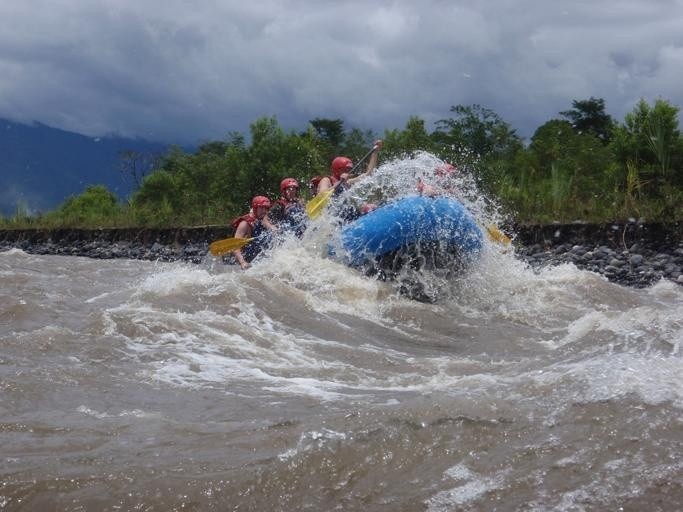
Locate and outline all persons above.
[229,139,461,272]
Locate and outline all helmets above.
[252,196,271,207]
[311,176,322,185]
[435,164,454,176]
[332,156,353,172]
[280,178,299,191]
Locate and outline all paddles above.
[456,193,512,247]
[211,221,305,256]
[306,145,378,221]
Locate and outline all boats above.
[241,194,484,304]
